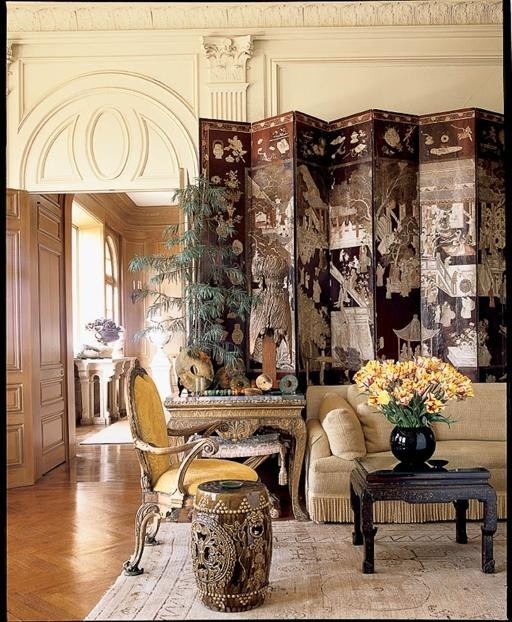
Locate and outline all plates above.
[427,460,449,470]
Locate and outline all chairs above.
[122,364,261,579]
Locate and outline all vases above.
[389,425,437,469]
[93,331,120,358]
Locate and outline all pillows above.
[318,389,391,461]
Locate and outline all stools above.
[192,433,286,518]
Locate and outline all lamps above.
[131,280,143,304]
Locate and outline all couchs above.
[307,385,507,524]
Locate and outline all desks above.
[349,456,495,575]
[76,359,136,424]
[165,389,307,525]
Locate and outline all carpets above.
[86,523,508,621]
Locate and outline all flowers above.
[88,317,121,343]
[352,358,475,426]
